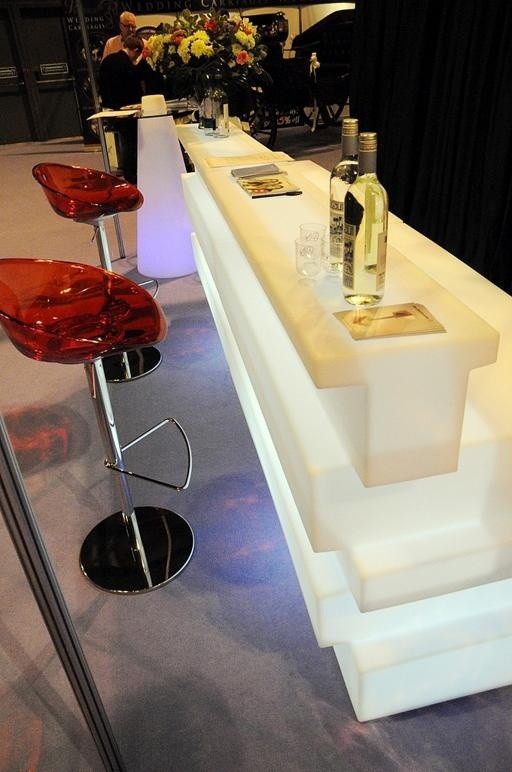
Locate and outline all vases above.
[193,89,215,134]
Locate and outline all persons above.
[98,36,151,184]
[103,12,150,179]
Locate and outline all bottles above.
[213,67,229,138]
[198,96,204,129]
[329,118,359,269]
[343,131,389,309]
[205,78,217,136]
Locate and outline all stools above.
[0,256,196,596]
[32,159,165,384]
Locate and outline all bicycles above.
[237,41,348,150]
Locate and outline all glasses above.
[120,22,136,28]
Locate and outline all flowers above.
[140,10,262,89]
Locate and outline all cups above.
[294,239,322,278]
[320,231,335,273]
[299,223,326,258]
